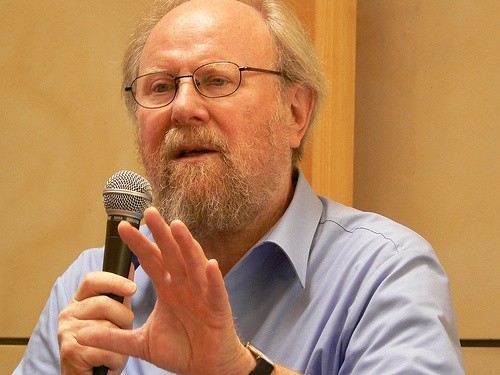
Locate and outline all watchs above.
[244,341,275,375]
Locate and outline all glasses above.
[125,61,282,109]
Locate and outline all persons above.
[10,1,467,375]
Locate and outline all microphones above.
[93,170,154,375]
